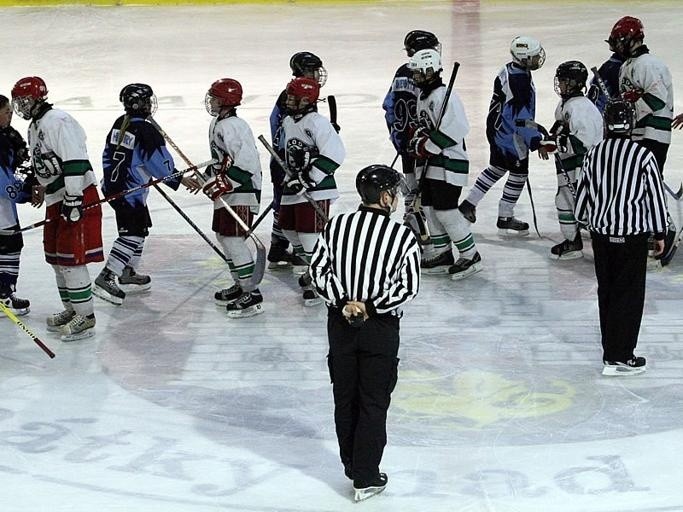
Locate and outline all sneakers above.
[458,198,477,224]
[448,249,482,275]
[297,270,321,304]
[60,311,96,336]
[0,293,30,311]
[214,283,245,302]
[421,248,455,268]
[226,286,264,311]
[602,355,647,368]
[352,471,389,490]
[94,265,127,300]
[266,240,306,266]
[118,265,151,286]
[551,230,584,255]
[496,216,530,231]
[46,308,77,327]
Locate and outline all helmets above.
[204,78,243,118]
[284,77,321,116]
[355,163,413,204]
[402,28,442,59]
[290,51,328,89]
[603,98,633,139]
[405,47,444,76]
[508,35,548,71]
[9,76,49,121]
[119,81,158,120]
[553,59,589,98]
[604,15,645,58]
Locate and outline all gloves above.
[201,171,235,203]
[60,191,83,225]
[539,135,560,155]
[281,169,318,198]
[401,121,439,160]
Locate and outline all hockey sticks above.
[0,158,219,236]
[408,62,460,242]
[526,178,541,238]
[147,115,266,285]
[662,181,682,200]
[514,118,576,203]
[242,95,340,241]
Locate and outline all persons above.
[573,99,669,368]
[402,46,481,275]
[10,76,104,336]
[308,164,421,489]
[382,30,443,246]
[539,16,683,267]
[0,94,41,311]
[277,79,346,300]
[93,83,201,299]
[184,78,264,312]
[266,52,341,267]
[458,35,546,231]
